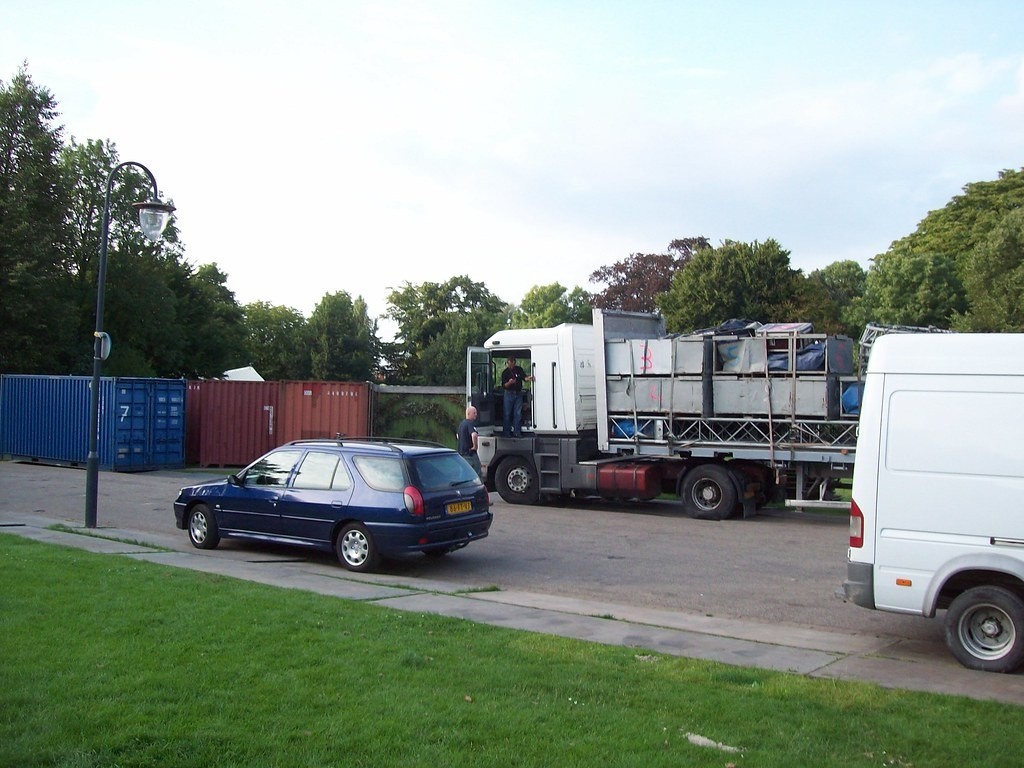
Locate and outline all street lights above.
[86,162,176,528]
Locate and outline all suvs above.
[173,434,490,571]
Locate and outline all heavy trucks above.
[464,306,867,519]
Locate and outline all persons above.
[456,406,493,506]
[501,357,535,438]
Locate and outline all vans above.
[834,332,1024,670]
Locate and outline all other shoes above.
[505,434,511,438]
[514,434,524,438]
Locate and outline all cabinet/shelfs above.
[606,322,866,418]
